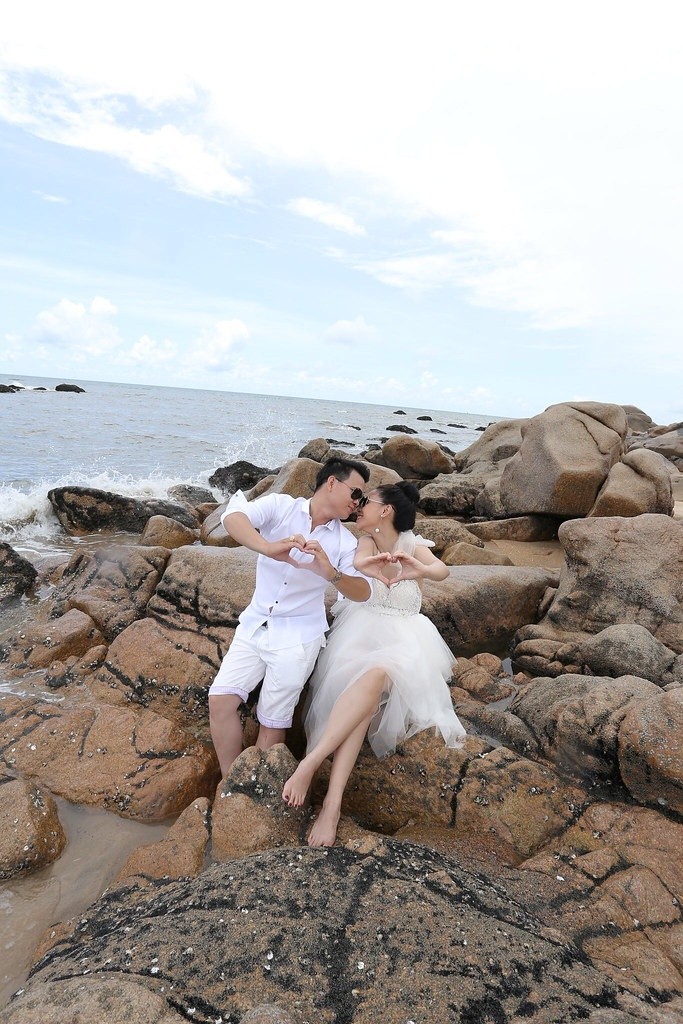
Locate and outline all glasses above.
[335,478,370,500]
[358,495,397,513]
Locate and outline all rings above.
[320,548,323,553]
[290,535,295,542]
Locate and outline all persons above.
[282,479,466,848]
[208,459,374,777]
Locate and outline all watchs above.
[328,568,341,583]
